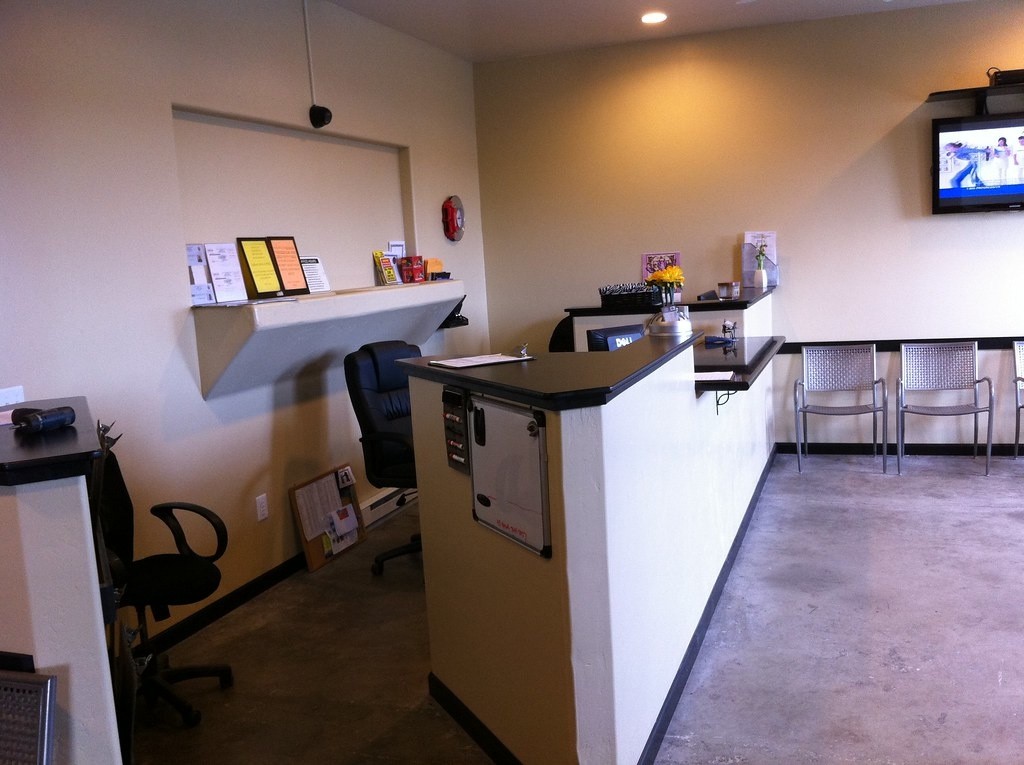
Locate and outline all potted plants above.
[659,308,679,320]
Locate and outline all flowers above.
[645,265,684,306]
[755,234,768,271]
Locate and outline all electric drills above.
[7,406,76,435]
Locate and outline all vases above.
[754,270,767,289]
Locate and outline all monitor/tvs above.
[932,111,1024,215]
[697,289,719,300]
[587,323,645,352]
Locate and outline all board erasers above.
[473,406,486,446]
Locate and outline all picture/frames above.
[642,250,679,284]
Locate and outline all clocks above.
[442,195,466,240]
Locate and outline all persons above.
[1013,136,1024,178]
[945,142,991,188]
[993,137,1011,185]
[983,146,990,161]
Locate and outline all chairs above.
[897,342,995,477]
[344,340,421,574]
[1013,341,1024,458]
[88,450,236,728]
[794,344,889,474]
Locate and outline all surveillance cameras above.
[309,104,332,128]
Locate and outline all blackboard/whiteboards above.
[463,391,555,559]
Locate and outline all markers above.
[447,438,465,451]
[447,452,465,463]
[442,412,463,423]
